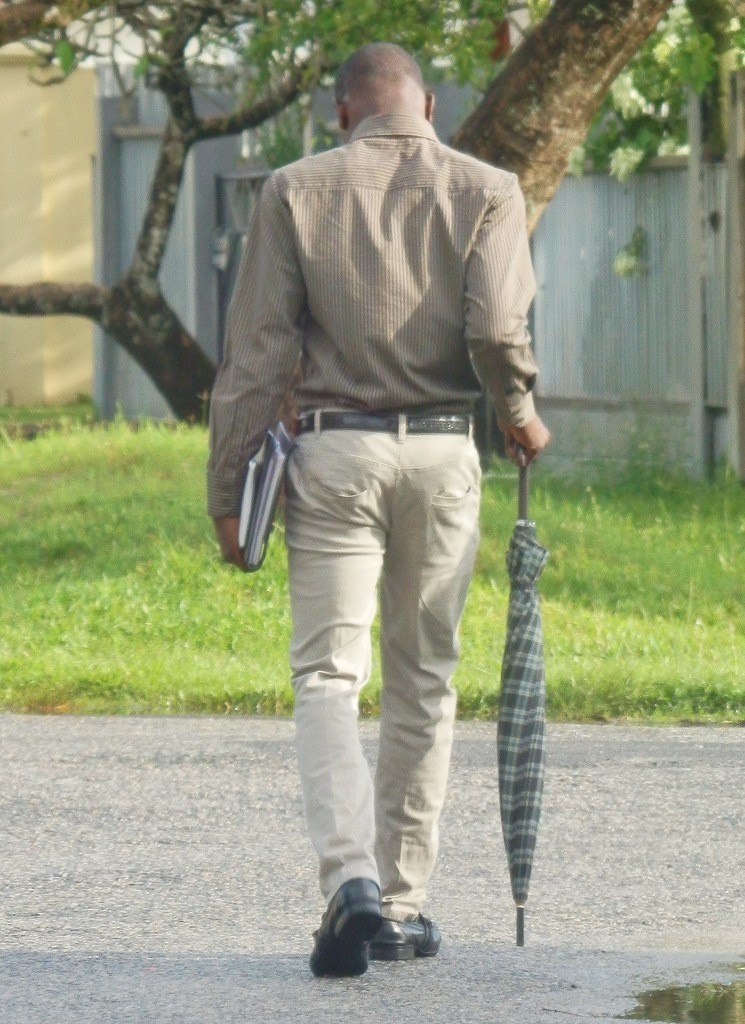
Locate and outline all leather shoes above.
[309,879,382,978]
[369,912,441,961]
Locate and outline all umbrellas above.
[496,436,546,946]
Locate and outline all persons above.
[205,43,552,978]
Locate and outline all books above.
[234,419,295,575]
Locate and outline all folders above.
[245,421,293,573]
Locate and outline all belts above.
[296,412,470,434]
